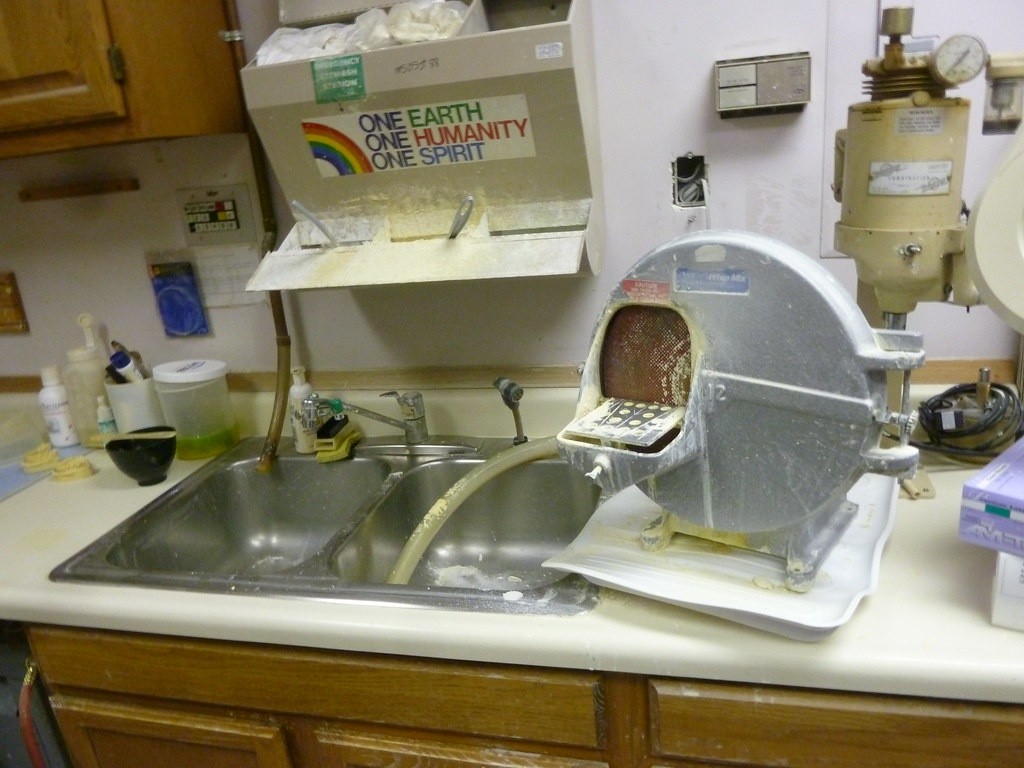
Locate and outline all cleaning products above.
[290,366,324,454]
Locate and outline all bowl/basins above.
[106,426,176,486]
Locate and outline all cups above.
[103,377,165,434]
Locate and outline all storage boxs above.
[991,551,1024,631]
[959,435,1024,556]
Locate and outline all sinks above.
[109,460,388,580]
[302,390,430,445]
[334,461,611,616]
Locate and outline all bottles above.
[152,358,238,461]
[96,396,117,434]
[38,367,78,447]
[62,312,106,447]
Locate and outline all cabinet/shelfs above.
[0,0,247,159]
[17,626,1024,768]
